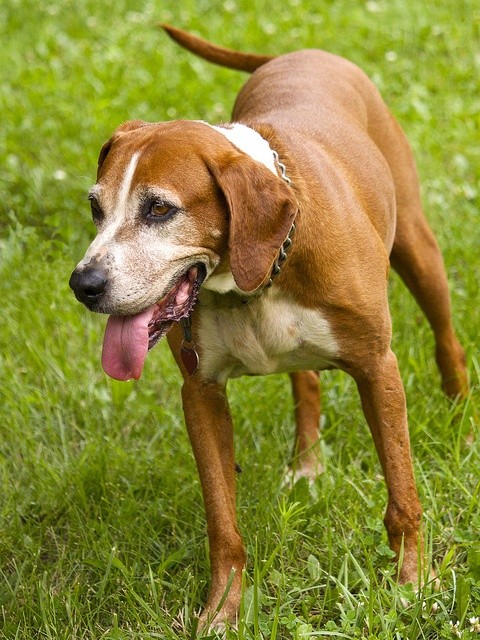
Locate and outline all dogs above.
[70,24,480,640]
